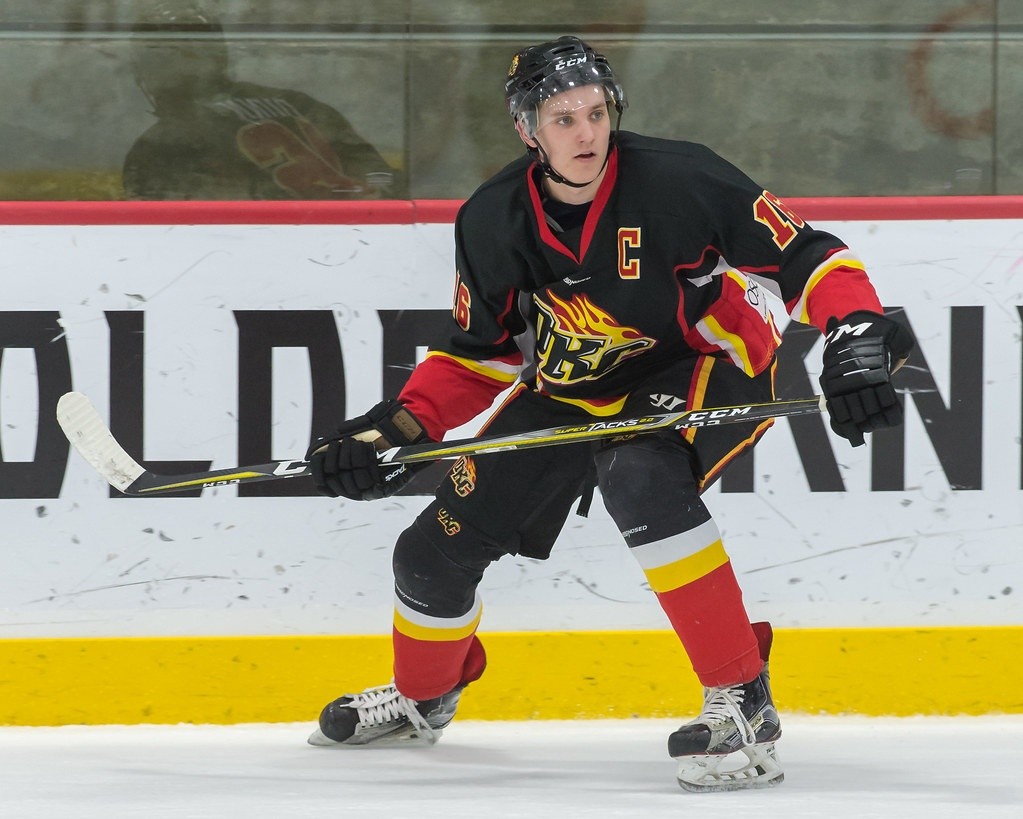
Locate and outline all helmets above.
[132,0,228,111]
[504,35,624,157]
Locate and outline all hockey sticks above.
[53,385,825,501]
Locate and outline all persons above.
[120,0,408,198]
[320,36,900,758]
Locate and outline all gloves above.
[818,309,914,447]
[305,396,440,501]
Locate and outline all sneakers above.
[306,634,486,748]
[667,621,784,793]
